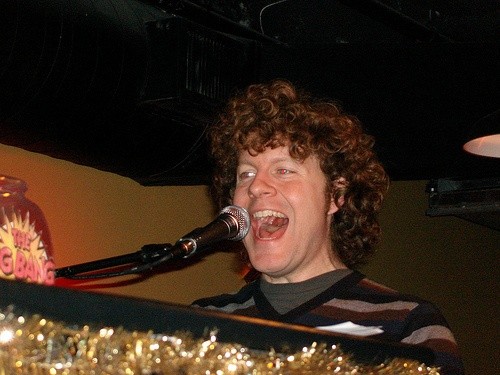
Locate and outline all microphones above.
[170,205,251,258]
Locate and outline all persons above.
[181,75,467,375]
